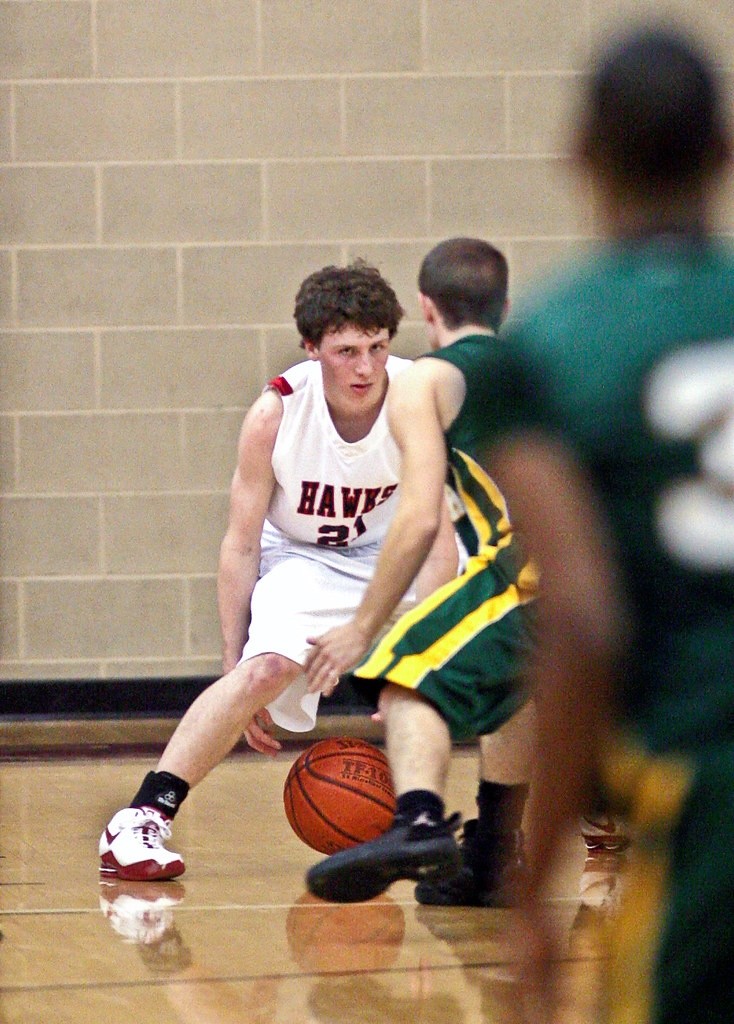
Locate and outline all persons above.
[90,877,625,1023]
[300,238,547,907]
[482,25,733,1024]
[95,258,633,880]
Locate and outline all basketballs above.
[279,737,400,855]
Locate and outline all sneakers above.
[98,806,185,882]
[99,881,186,947]
[305,811,463,903]
[415,820,529,909]
[570,816,638,946]
